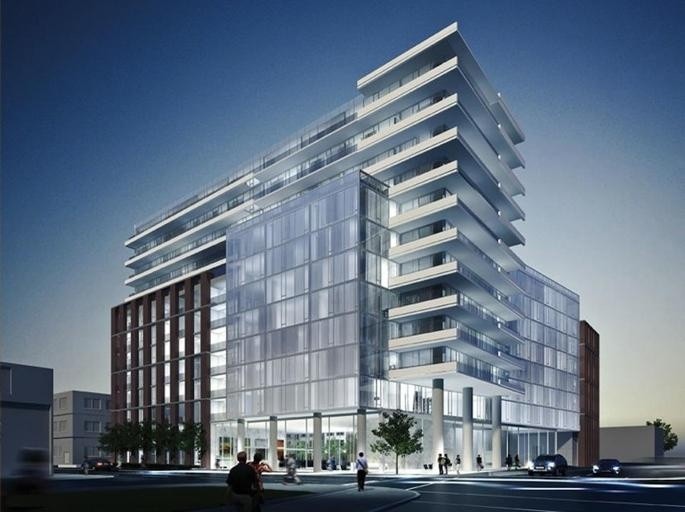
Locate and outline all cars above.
[590,458,624,476]
[78,457,117,475]
[524,454,571,476]
